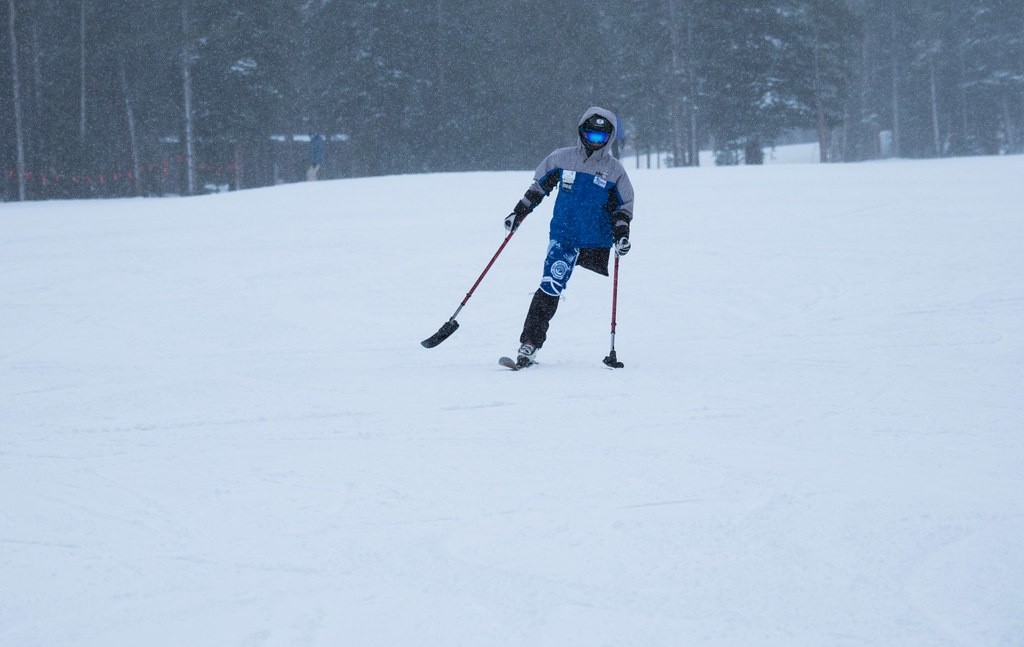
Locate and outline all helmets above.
[580,115,613,150]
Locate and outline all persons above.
[502,106,635,365]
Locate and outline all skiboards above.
[498,355,538,371]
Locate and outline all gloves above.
[503,210,525,233]
[614,237,631,257]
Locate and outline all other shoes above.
[517,341,539,363]
